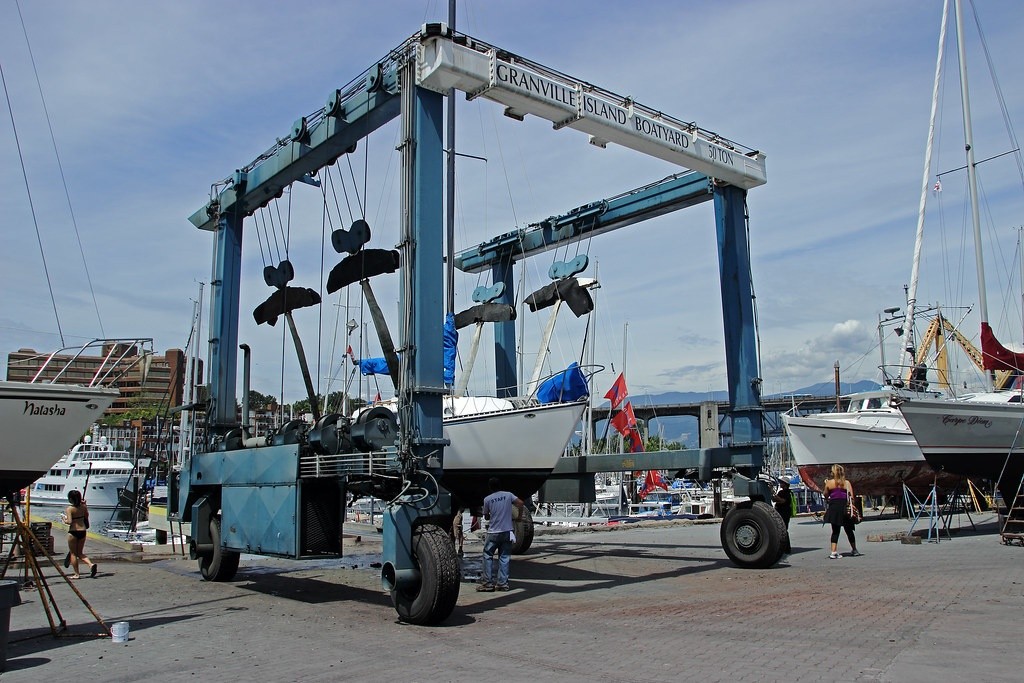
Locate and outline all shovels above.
[470,507,543,525]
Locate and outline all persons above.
[62,490,97,578]
[772,482,794,530]
[475,477,523,592]
[453,510,464,557]
[9,501,26,523]
[821,464,860,558]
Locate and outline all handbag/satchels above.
[846,504,862,524]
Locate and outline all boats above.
[775,304,980,503]
[20,431,146,509]
[1,332,158,507]
[537,481,714,520]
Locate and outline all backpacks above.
[788,490,796,516]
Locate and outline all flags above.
[603,371,628,409]
[639,470,668,498]
[610,400,637,438]
[629,431,648,477]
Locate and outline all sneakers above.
[851,549,859,555]
[829,552,842,559]
[476,583,495,592]
[494,584,509,591]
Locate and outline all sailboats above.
[883,0,1024,503]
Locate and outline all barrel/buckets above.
[111,622,129,643]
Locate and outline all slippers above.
[90,564,97,577]
[69,575,80,579]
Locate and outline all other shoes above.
[458,549,463,556]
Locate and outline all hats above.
[777,477,790,487]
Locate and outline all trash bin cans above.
[851,497,863,517]
[0,580,18,672]
[156,529,167,544]
